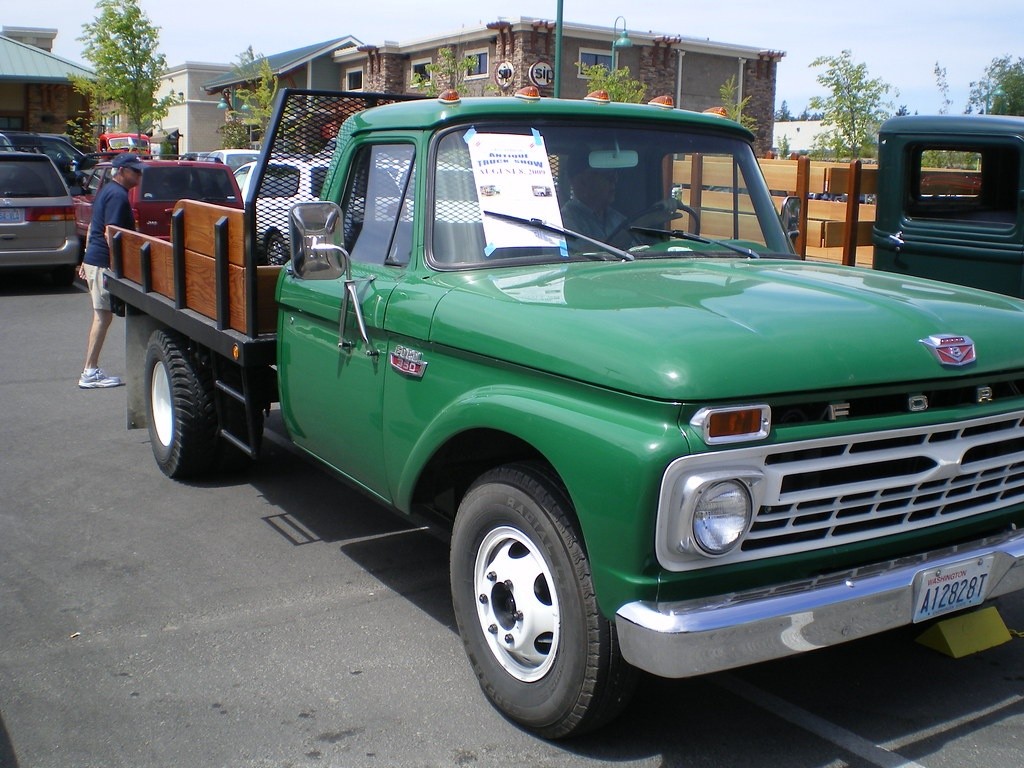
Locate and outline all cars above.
[378,161,482,224]
[0,150,81,286]
[187,149,261,191]
[232,158,407,266]
[99,133,151,161]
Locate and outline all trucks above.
[102,86,1024,743]
[671,115,1024,302]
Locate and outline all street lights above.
[612,16,632,70]
[217,89,250,120]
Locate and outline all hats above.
[112,153,150,170]
[567,134,622,178]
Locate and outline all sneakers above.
[78,368,121,388]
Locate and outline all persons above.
[79,153,152,388]
[542,137,684,255]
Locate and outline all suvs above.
[1,130,93,195]
[70,154,244,241]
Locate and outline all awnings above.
[150,128,178,143]
